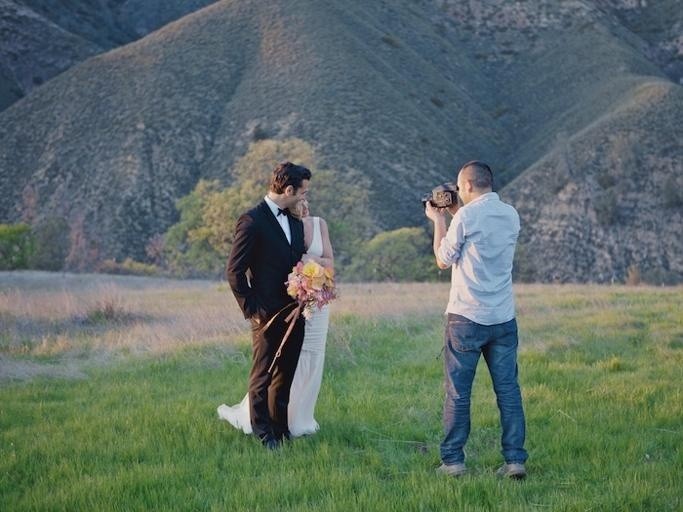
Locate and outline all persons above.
[217,198,334,439]
[226,160,312,453]
[425,160,529,477]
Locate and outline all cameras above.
[422,184,458,209]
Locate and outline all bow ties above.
[276,207,290,217]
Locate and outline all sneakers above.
[496,462,528,479]
[437,462,469,476]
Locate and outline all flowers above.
[284,257,339,322]
